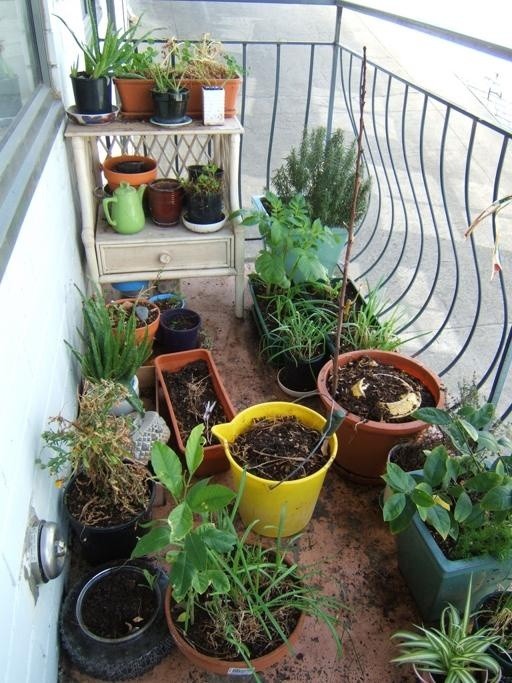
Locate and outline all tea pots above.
[102,180,149,234]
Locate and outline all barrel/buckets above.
[211,393,338,539]
